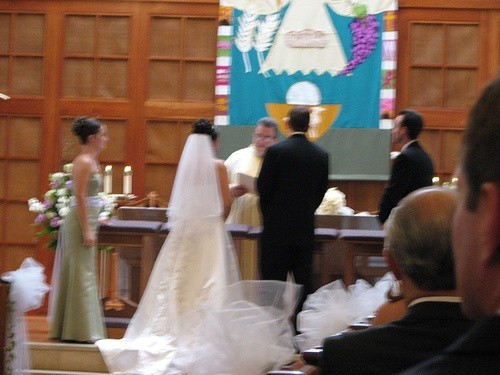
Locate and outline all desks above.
[99,219,384,307]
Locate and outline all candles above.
[124,166,132,195]
[103,164,113,195]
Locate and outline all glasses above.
[252,133,277,142]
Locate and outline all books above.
[237,157,263,196]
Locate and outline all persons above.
[48,119,107,342]
[223,118,279,305]
[300,76,500,375]
[254,106,330,354]
[161,120,234,342]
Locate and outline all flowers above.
[28,163,119,253]
